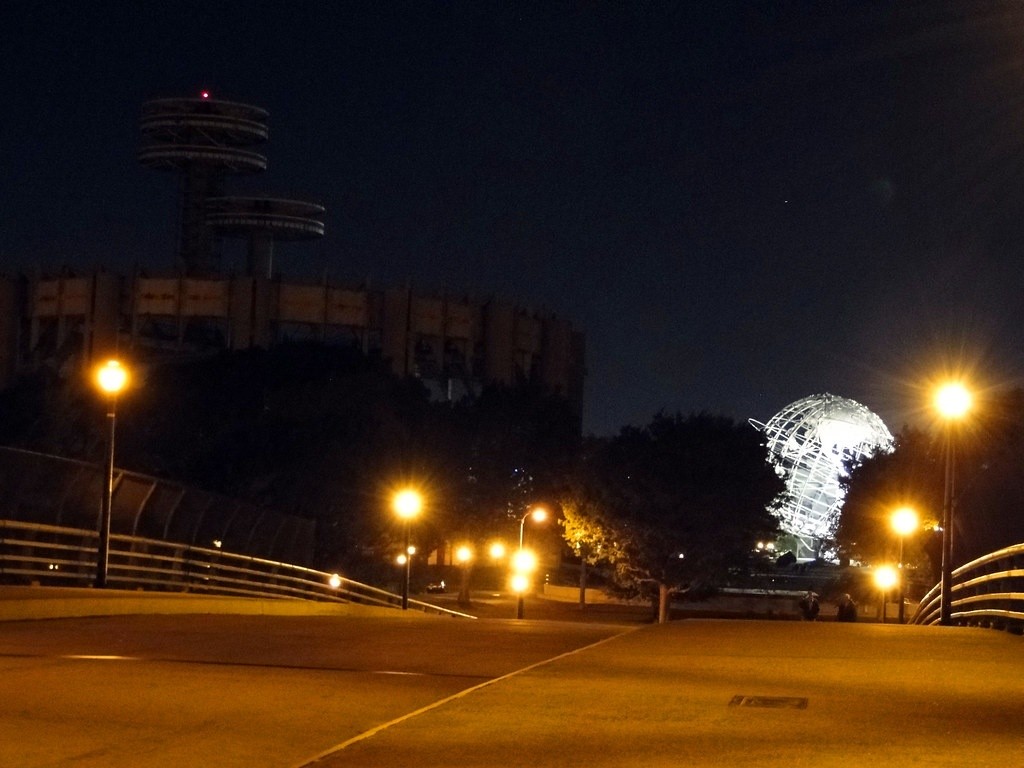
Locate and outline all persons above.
[834,593,857,623]
[799,590,820,621]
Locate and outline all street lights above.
[877,566,897,623]
[934,381,971,629]
[93,359,129,588]
[891,507,918,625]
[394,488,420,610]
[516,506,547,621]
